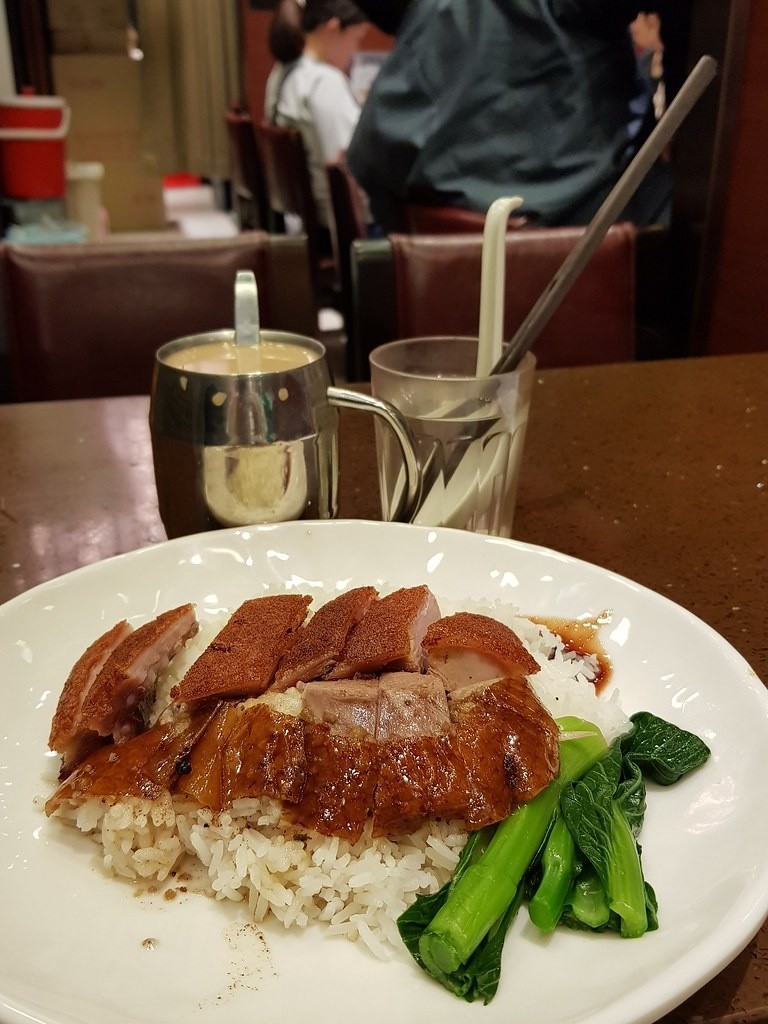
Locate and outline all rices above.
[45,581,638,957]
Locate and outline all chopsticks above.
[393,56,717,522]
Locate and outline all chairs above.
[224,109,363,232]
[0,233,281,403]
[389,218,641,374]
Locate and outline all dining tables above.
[0,350,768,1024]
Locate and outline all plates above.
[0,520,768,1024]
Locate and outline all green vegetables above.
[395,706,707,1007]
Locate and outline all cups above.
[363,333,537,543]
[66,158,103,248]
[151,324,423,546]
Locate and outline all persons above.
[258,0,703,384]
